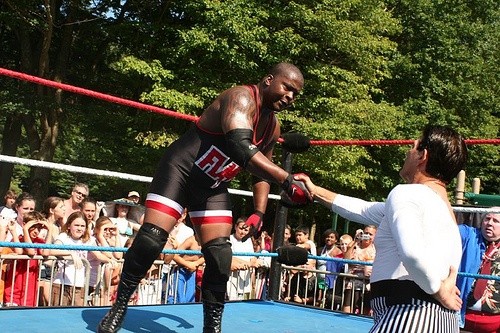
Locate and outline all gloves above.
[281,173,314,205]
[241,211,265,242]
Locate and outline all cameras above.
[357,233,370,241]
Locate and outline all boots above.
[98,279,137,332]
[202,301,224,333]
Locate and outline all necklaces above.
[427,181,446,188]
[482,250,499,261]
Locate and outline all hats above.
[112,198,140,208]
[128,191,140,197]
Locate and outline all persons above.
[98,63,314,333]
[0,183,500,333]
[288,126,466,333]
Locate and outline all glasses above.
[75,191,86,198]
